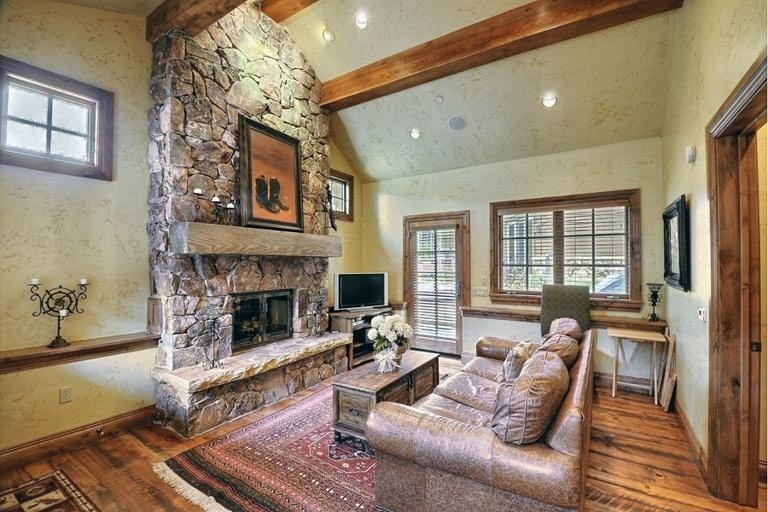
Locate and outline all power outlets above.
[58,385,73,407]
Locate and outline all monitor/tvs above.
[333,271,389,312]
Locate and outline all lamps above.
[645,282,665,322]
[538,87,557,112]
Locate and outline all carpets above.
[152,346,468,512]
[0,468,104,511]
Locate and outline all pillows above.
[490,348,570,447]
[364,326,596,511]
[546,316,583,341]
[495,337,530,385]
[533,333,579,370]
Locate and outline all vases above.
[387,340,412,372]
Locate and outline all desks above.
[605,327,666,406]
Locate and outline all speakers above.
[353,329,372,343]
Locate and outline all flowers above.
[366,316,412,373]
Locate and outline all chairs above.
[539,283,591,339]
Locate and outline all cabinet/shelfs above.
[231,289,295,354]
[327,306,408,370]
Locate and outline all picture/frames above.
[659,194,690,293]
[236,113,304,233]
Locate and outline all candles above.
[59,309,69,321]
[78,278,89,289]
[30,275,41,286]
